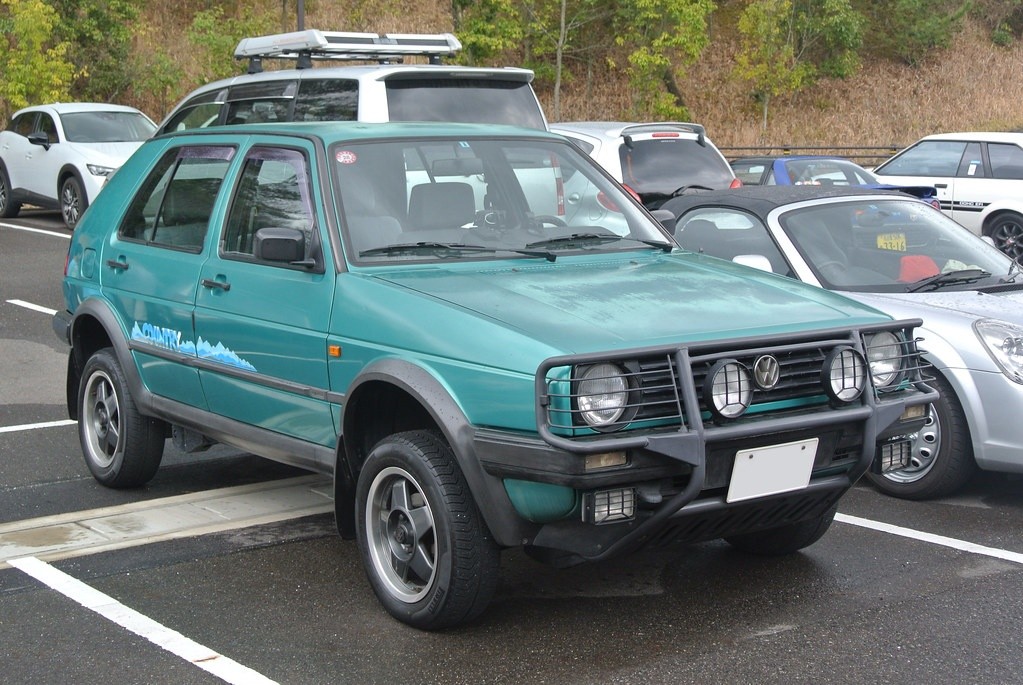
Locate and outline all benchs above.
[685,220,790,272]
[163,179,304,257]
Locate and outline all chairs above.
[407,182,477,244]
[344,170,403,254]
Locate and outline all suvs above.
[794,131,1023,265]
[50,119,941,631]
[101,28,565,225]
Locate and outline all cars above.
[0,101,160,230]
[728,154,940,251]
[546,121,752,236]
[628,185,1023,500]
